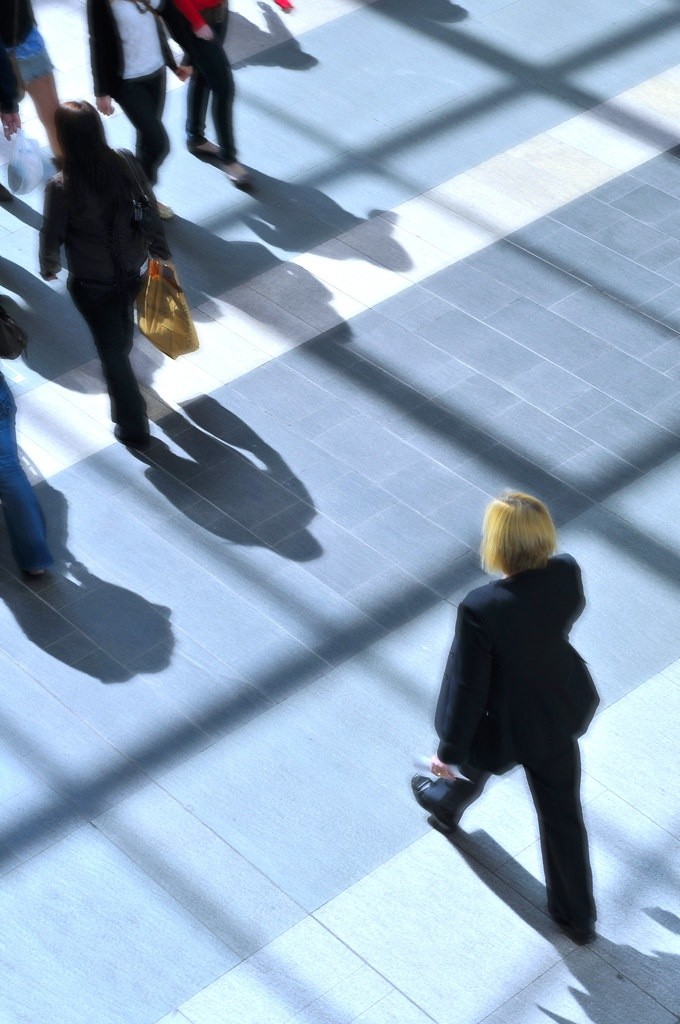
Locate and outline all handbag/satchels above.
[3,121,43,197]
[0,307,28,361]
[134,259,199,359]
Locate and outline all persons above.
[0,0,260,579]
[411,490,600,945]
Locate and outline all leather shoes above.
[548,901,596,946]
[412,774,457,832]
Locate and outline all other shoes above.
[113,425,150,451]
[187,140,223,155]
[157,204,173,221]
[224,163,249,187]
[26,568,46,577]
